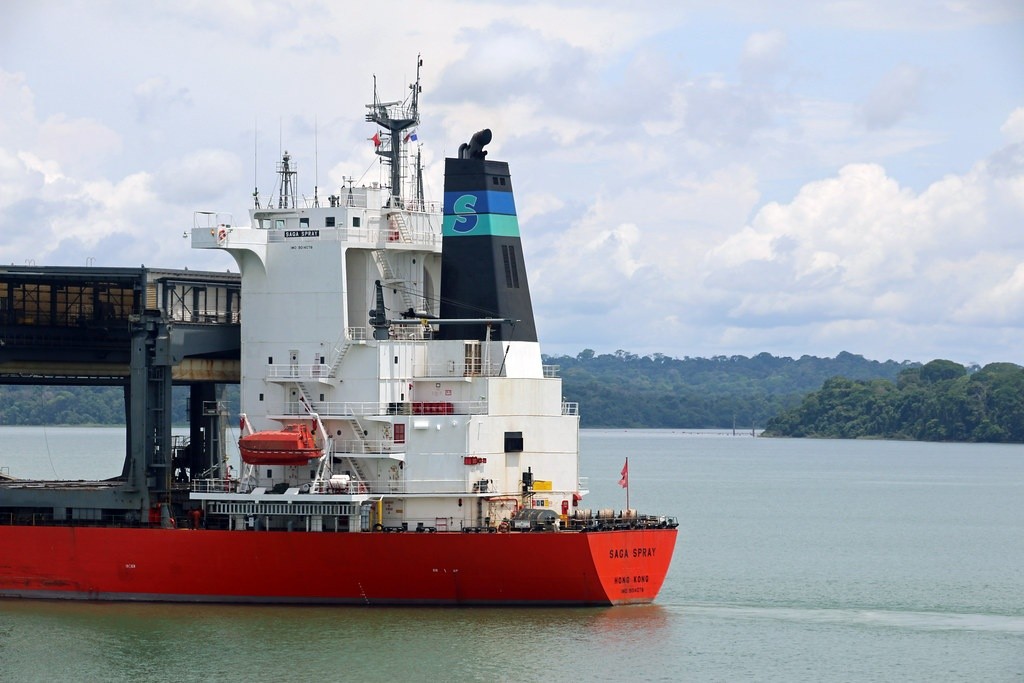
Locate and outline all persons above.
[188,507,201,530]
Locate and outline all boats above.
[0,54,679,606]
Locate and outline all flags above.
[371,133,379,147]
[618,461,628,489]
[403,129,417,144]
